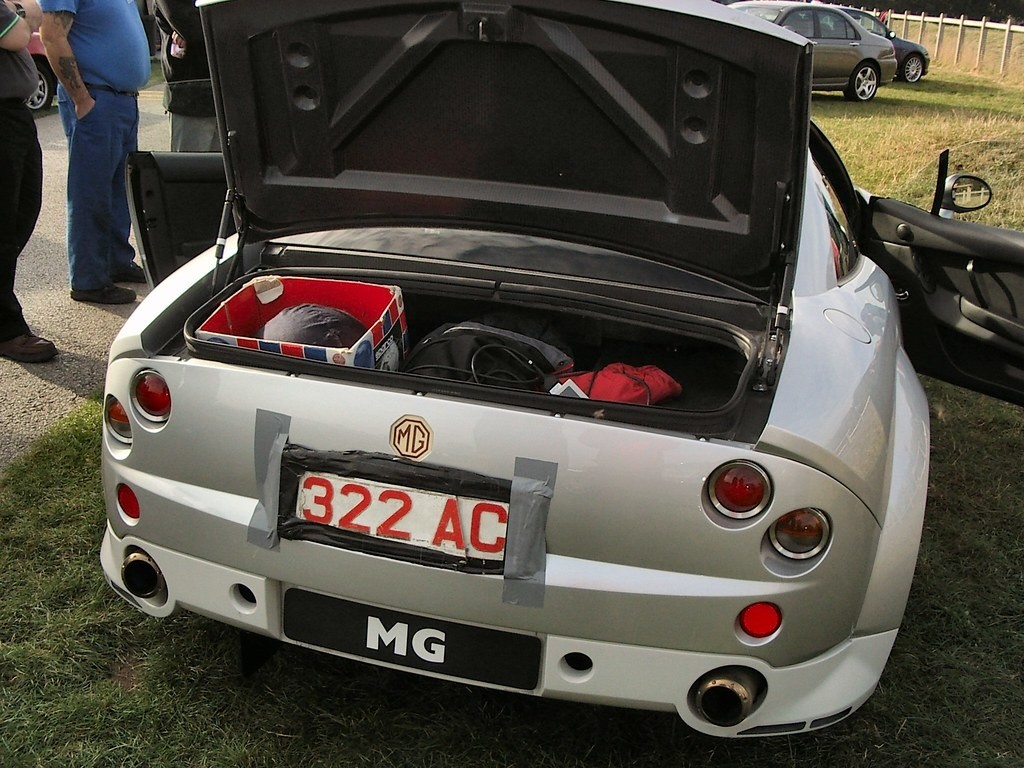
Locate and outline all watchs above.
[14,2,25,18]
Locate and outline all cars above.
[804,5,931,83]
[26,30,59,111]
[725,1,898,101]
[98,0,1024,740]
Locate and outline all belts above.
[0,97,31,105]
[85,83,139,97]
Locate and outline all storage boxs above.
[195,275,409,373]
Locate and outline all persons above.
[0,0,56,364]
[152,0,222,150]
[38,0,150,304]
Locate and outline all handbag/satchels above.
[401,321,573,395]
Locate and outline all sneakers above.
[71,283,136,303]
[0,331,59,363]
[109,262,146,283]
